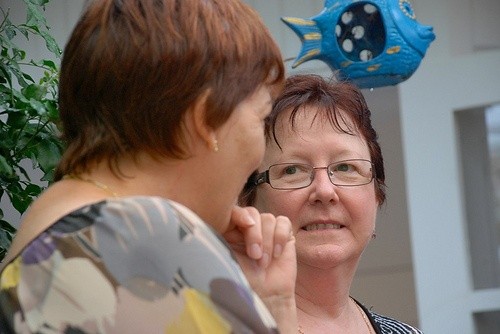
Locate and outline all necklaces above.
[61,174,124,199]
[297,297,372,333]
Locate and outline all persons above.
[240,69,423,334]
[0,0,298,333]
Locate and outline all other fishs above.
[280,0,435,89]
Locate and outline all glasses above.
[255,159,377,190]
